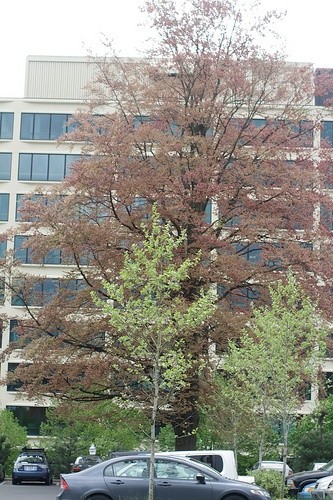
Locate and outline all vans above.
[117,450,238,483]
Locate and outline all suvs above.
[11,447,54,485]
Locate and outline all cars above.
[284,458,333,498]
[70,455,103,473]
[56,454,270,500]
[251,461,294,477]
[298,475,333,500]
